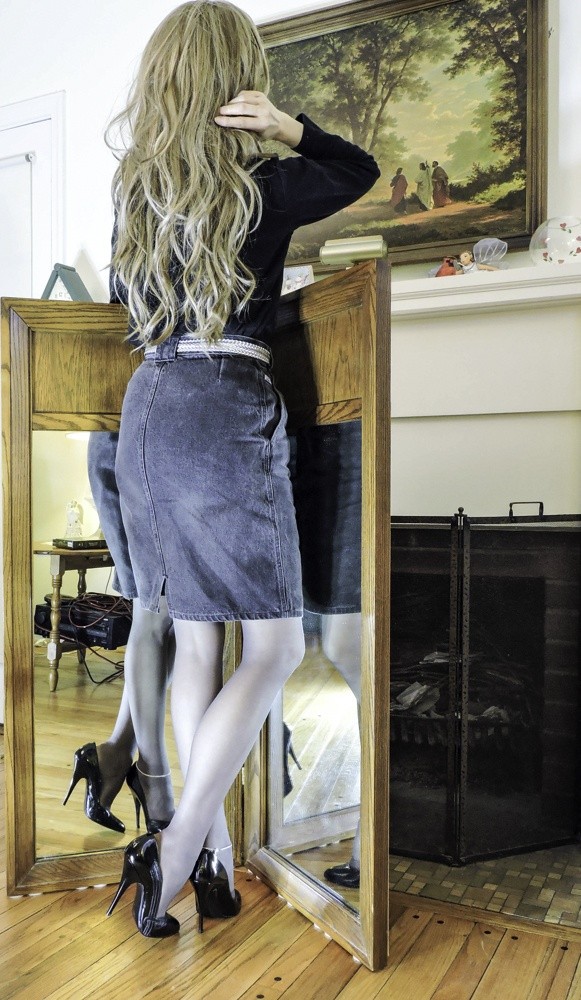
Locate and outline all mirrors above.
[29,414,233,864]
[266,619,361,855]
[251,413,366,915]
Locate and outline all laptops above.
[53,537,110,549]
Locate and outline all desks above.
[33,540,111,692]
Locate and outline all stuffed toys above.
[455,249,500,276]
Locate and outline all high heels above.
[126,760,170,834]
[104,834,180,936]
[323,867,360,886]
[63,742,126,833]
[193,848,242,934]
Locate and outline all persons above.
[62,430,176,834]
[106,0,380,938]
[283,423,363,887]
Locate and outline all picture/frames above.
[157,1,550,273]
